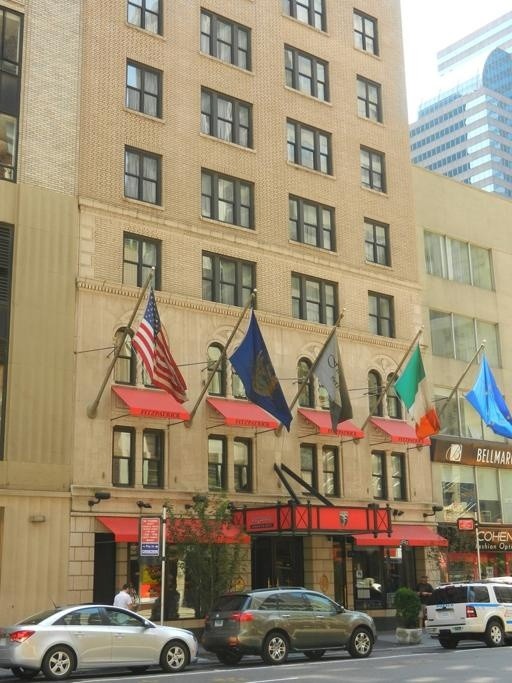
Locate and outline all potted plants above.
[394,587,422,646]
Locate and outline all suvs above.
[423,576,512,648]
[202,586,377,667]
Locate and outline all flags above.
[226,309,295,433]
[313,323,354,433]
[395,343,441,441]
[462,356,511,439]
[133,292,188,402]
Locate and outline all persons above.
[112,583,135,612]
[148,598,168,620]
[481,571,486,578]
[163,583,180,618]
[415,575,434,629]
[127,582,140,611]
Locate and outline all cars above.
[0,598,201,680]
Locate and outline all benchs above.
[57,612,111,625]
